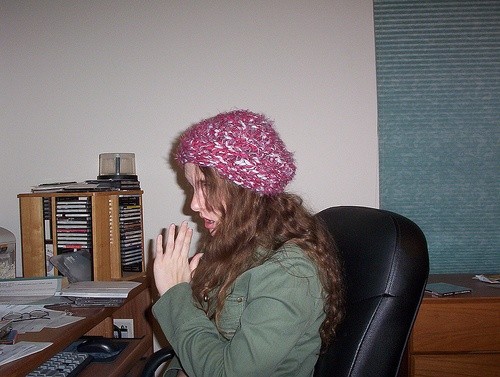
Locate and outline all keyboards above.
[25,352,92,377]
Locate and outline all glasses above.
[1,310,51,322]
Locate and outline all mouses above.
[76,338,117,354]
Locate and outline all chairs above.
[142,206,429,377]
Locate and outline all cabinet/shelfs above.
[0,276,154,377]
[406,273,500,377]
[17,190,145,282]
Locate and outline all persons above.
[153,110,346,377]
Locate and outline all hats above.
[171,110,296,197]
